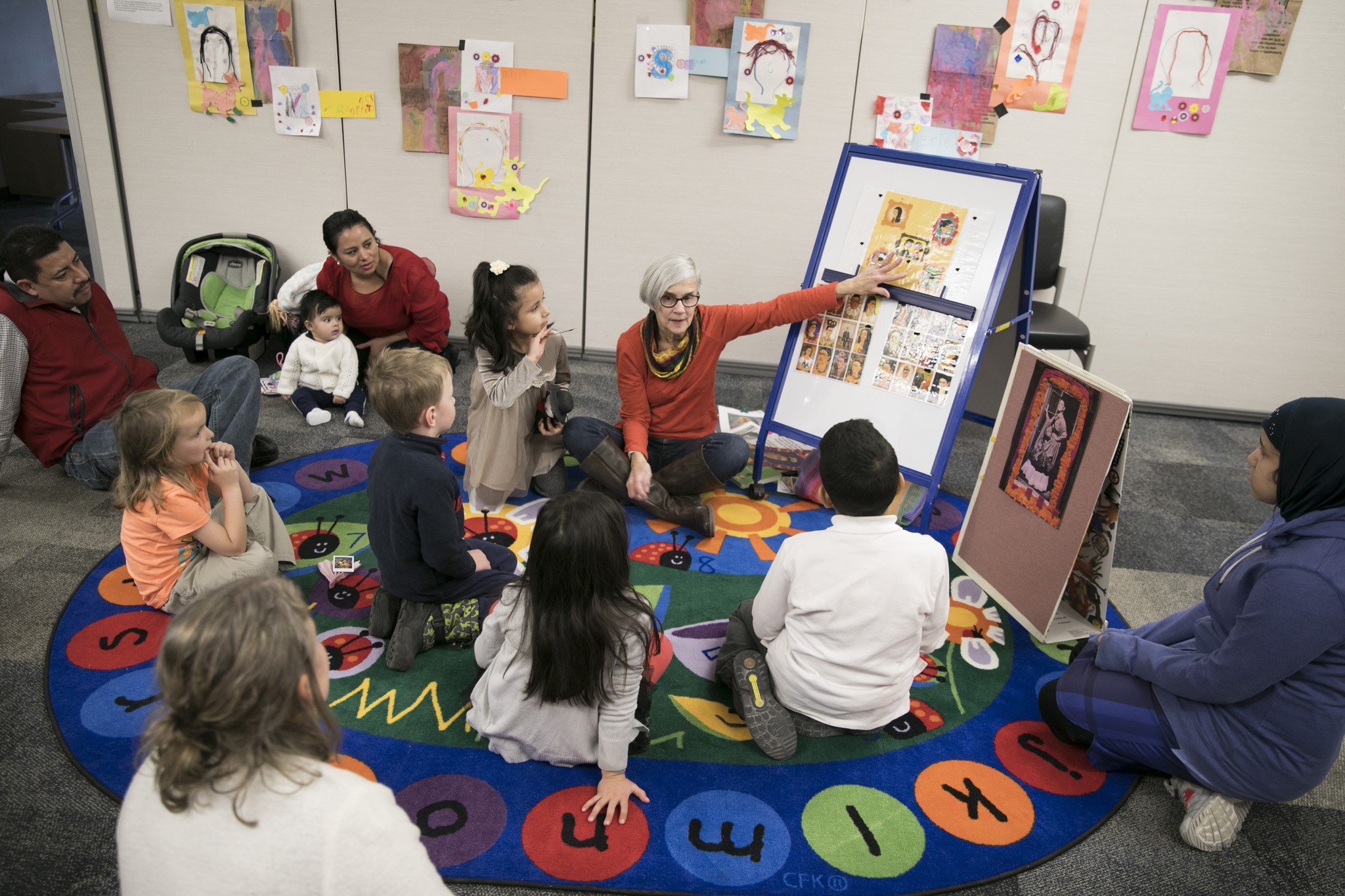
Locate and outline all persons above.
[466,490,662,825]
[714,419,950,759]
[116,578,453,896]
[278,289,366,427]
[367,346,519,670]
[563,249,907,538]
[316,208,451,390]
[0,225,279,491]
[1040,397,1345,852]
[465,261,571,506]
[1021,399,1067,492]
[114,388,295,613]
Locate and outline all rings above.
[878,263,882,268]
[871,262,875,265]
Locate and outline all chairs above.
[1028,194,1096,373]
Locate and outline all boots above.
[385,596,488,671]
[653,445,726,496]
[579,435,715,538]
[368,582,403,639]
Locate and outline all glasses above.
[659,292,700,307]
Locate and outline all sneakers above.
[251,435,278,467]
[734,650,797,760]
[1163,777,1254,853]
[789,708,883,738]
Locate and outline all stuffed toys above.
[535,385,574,435]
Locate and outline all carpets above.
[48,432,1142,896]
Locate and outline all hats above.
[1261,398,1298,451]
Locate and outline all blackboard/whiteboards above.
[770,142,1044,486]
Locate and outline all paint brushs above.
[544,328,576,337]
